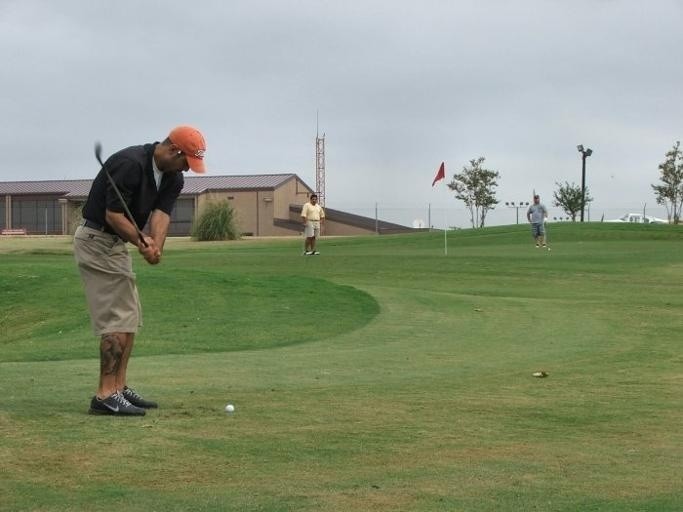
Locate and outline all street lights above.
[578,145,593,222]
[504,201,529,223]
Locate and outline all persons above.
[299,194,326,255]
[70,124,208,418]
[526,194,549,249]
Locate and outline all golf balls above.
[548,247,552,250]
[226,403,234,412]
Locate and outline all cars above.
[605,214,667,224]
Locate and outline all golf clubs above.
[95,143,149,248]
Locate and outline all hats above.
[168,125,206,174]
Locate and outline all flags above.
[431,162,446,187]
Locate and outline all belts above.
[79,218,115,234]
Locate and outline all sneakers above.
[123,386,158,408]
[305,250,320,255]
[536,243,546,248]
[88,392,145,416]
